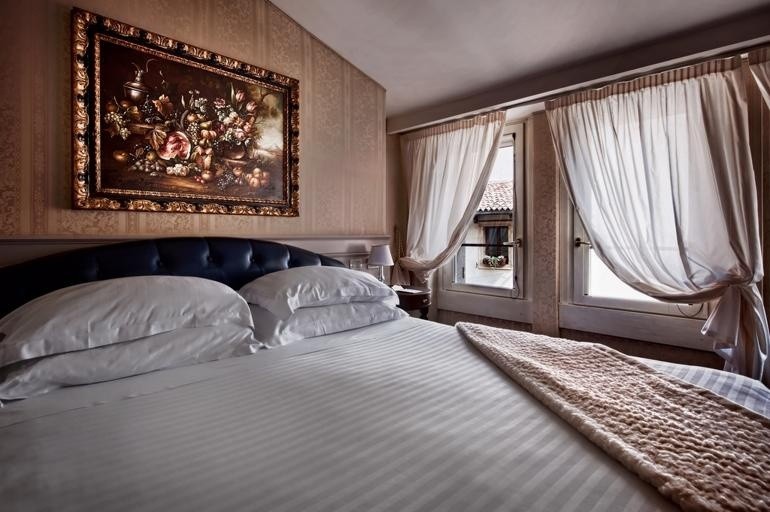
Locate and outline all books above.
[397,287,424,294]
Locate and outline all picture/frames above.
[69,2,302,219]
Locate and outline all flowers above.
[139,82,259,177]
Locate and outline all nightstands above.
[388,284,432,320]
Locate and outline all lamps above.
[365,243,398,286]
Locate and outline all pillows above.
[249,299,410,351]
[0,323,264,409]
[0,274,257,368]
[237,266,397,323]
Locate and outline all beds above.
[0,233,770,512]
